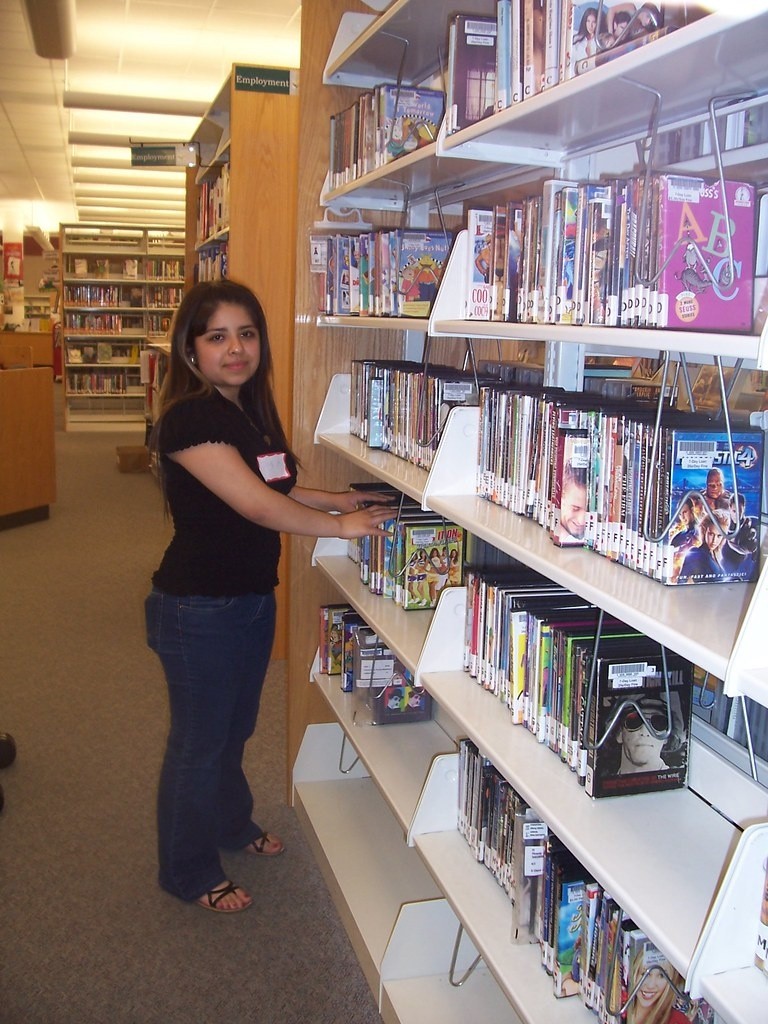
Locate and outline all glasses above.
[623,709,668,732]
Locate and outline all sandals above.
[248,831,285,856]
[196,879,254,911]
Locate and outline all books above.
[305,2,768,1024]
[0,152,236,433]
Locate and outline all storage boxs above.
[116,446,147,475]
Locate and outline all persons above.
[668,491,703,552]
[676,508,730,584]
[408,550,418,605]
[415,548,429,607]
[447,537,460,586]
[142,279,401,912]
[604,689,685,773]
[434,546,450,602]
[571,7,606,60]
[558,459,587,542]
[422,547,446,607]
[573,1,659,40]
[719,492,758,578]
[631,943,692,1024]
[596,11,632,52]
[698,466,735,520]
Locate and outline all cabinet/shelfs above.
[59,222,186,434]
[0,293,55,532]
[284,1,768,1024]
[184,63,300,663]
[144,336,172,429]
